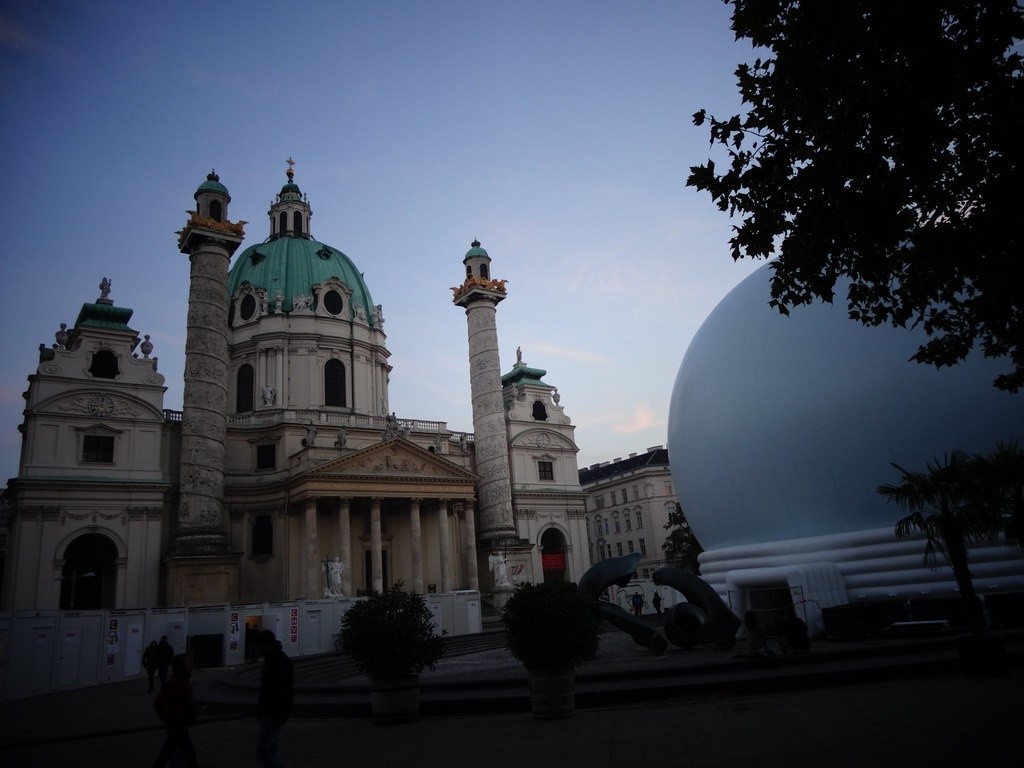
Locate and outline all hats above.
[256,630,276,648]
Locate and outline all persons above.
[385,412,398,436]
[516,346,522,362]
[653,591,663,617]
[55,323,69,345]
[99,277,113,298]
[142,635,174,694]
[632,592,644,616]
[338,425,349,448]
[497,551,510,585]
[141,334,153,355]
[263,384,276,404]
[154,652,201,768]
[332,557,341,596]
[304,420,318,446]
[254,629,296,764]
[433,436,442,449]
